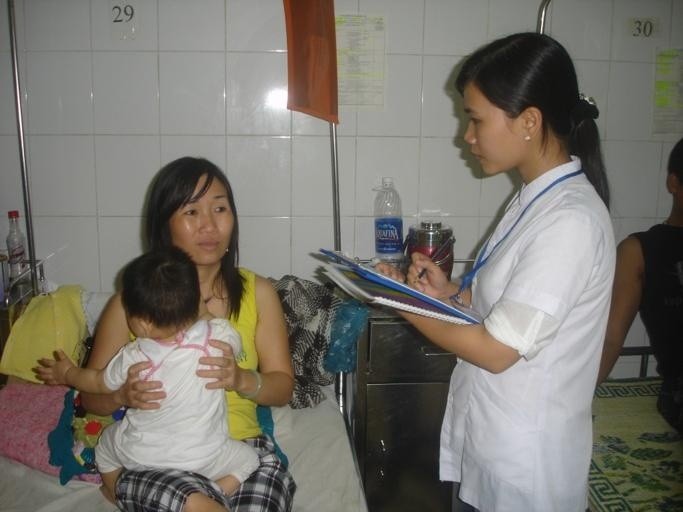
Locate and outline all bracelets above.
[236,369,263,400]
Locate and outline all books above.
[305,251,473,327]
[316,243,482,326]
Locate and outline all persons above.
[74,153,300,512]
[593,135,683,438]
[29,245,265,507]
[367,30,619,511]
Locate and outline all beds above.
[584,343,681,510]
[0,292,368,512]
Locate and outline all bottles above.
[372,176,404,267]
[6,210,29,285]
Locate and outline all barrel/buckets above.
[402,220,455,284]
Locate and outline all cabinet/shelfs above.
[329,286,457,511]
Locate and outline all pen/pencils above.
[413,236,453,283]
